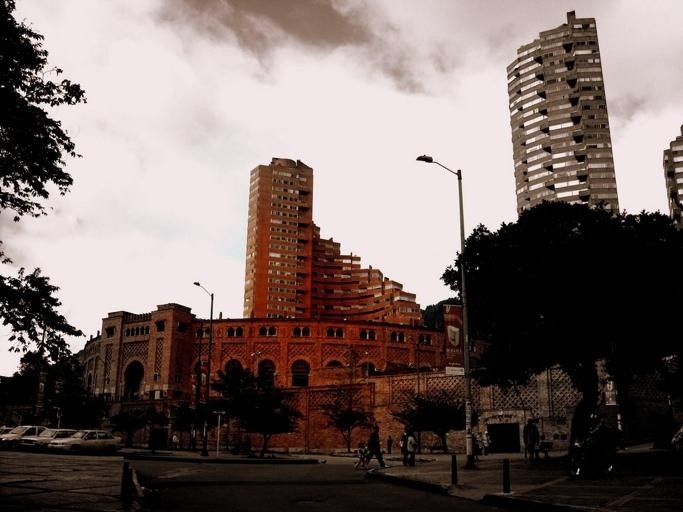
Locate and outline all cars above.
[0,425,123,456]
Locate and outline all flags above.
[441,303,466,376]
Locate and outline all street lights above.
[411,151,474,462]
[210,408,225,452]
[190,280,217,457]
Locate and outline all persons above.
[523,419,541,462]
[353,418,492,471]
[169,430,251,456]
